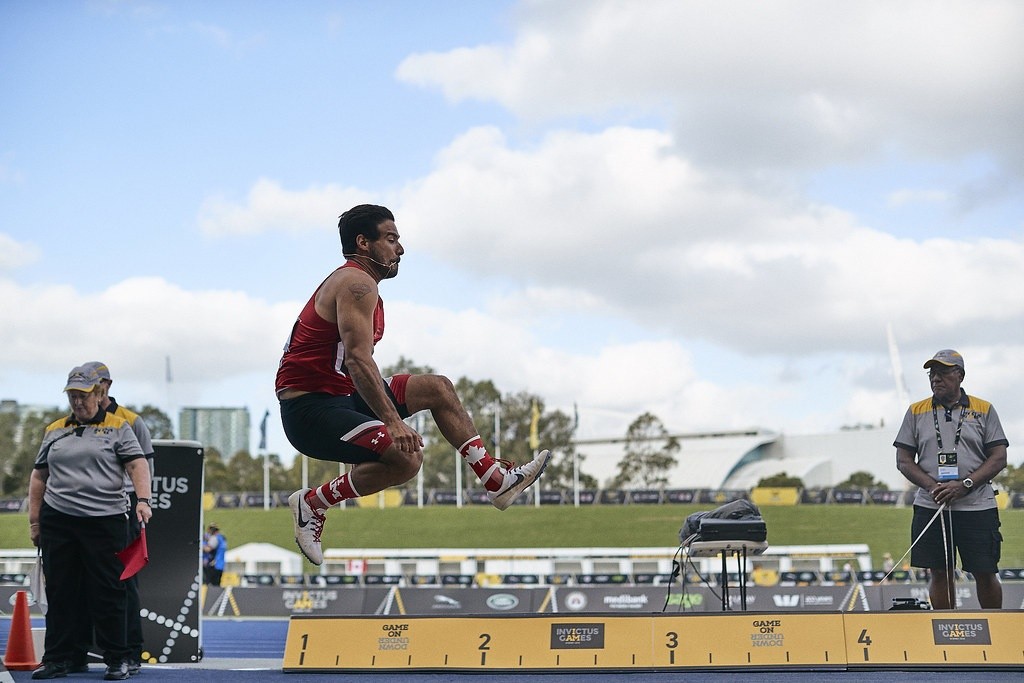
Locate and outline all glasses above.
[927,369,957,378]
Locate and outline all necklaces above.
[343,254,397,286]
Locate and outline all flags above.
[528,405,538,450]
[118,529,149,581]
[10,557,48,616]
[259,413,269,448]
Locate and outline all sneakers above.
[288,488,325,566]
[486,449,551,512]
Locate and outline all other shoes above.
[128,659,140,674]
[103,664,129,680]
[31,656,66,679]
[65,660,88,672]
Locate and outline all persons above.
[893,349,1010,608]
[29,362,155,680]
[941,456,946,463]
[202,523,225,587]
[275,204,549,567]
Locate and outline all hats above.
[62,367,100,392]
[82,362,111,381]
[924,349,964,370]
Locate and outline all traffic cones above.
[2,591,42,672]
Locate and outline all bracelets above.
[30,523,39,527]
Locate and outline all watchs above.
[962,478,973,492]
[137,498,151,506]
